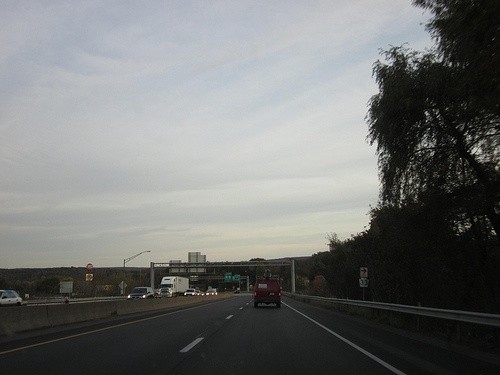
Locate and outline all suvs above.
[251,278,281,308]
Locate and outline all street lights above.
[123,250,151,295]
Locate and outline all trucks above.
[158,276,189,297]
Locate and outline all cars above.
[0,289,24,307]
[127,286,154,300]
[184,287,218,296]
[158,288,173,298]
